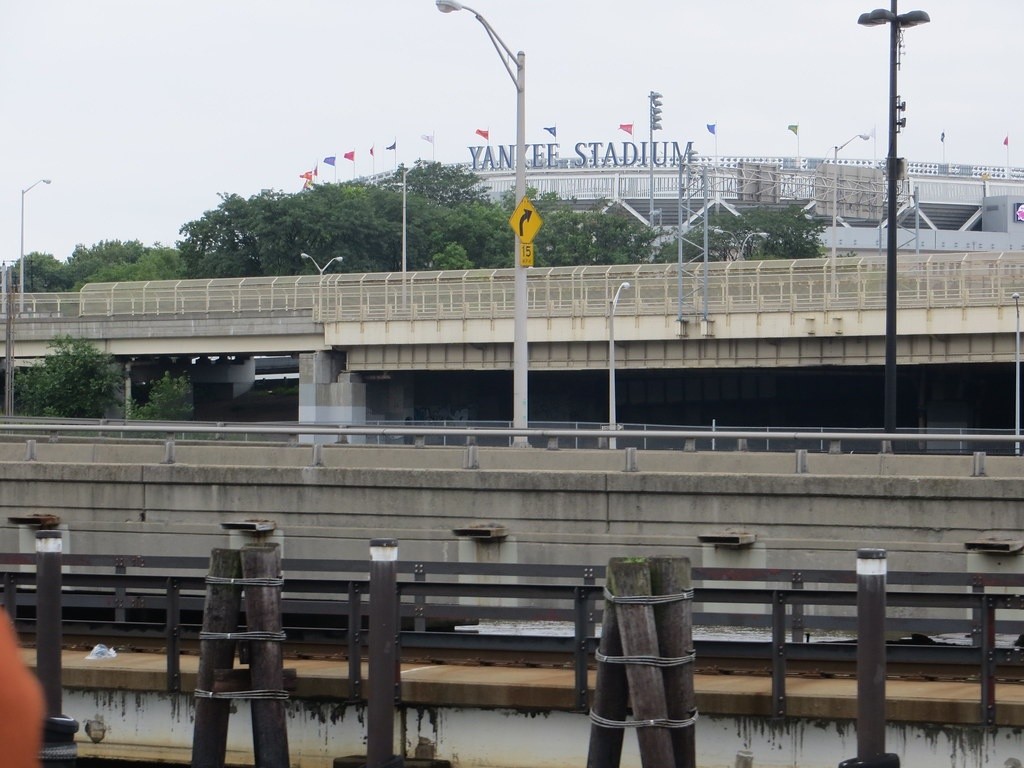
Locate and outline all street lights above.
[436,0,527,447]
[857,11,931,433]
[1012,291,1023,455]
[649,91,664,229]
[20,178,51,316]
[397,165,407,312]
[688,150,699,233]
[607,280,629,448]
[716,229,768,305]
[300,253,344,324]
[831,132,870,299]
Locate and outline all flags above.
[788,125,797,135]
[1003,137,1008,145]
[324,157,336,166]
[707,124,716,135]
[370,147,374,156]
[941,132,945,142]
[386,142,396,150]
[344,151,354,161]
[543,127,557,137]
[620,125,633,135]
[422,134,434,144]
[300,167,317,188]
[476,129,488,140]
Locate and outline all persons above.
[404,416,414,444]
